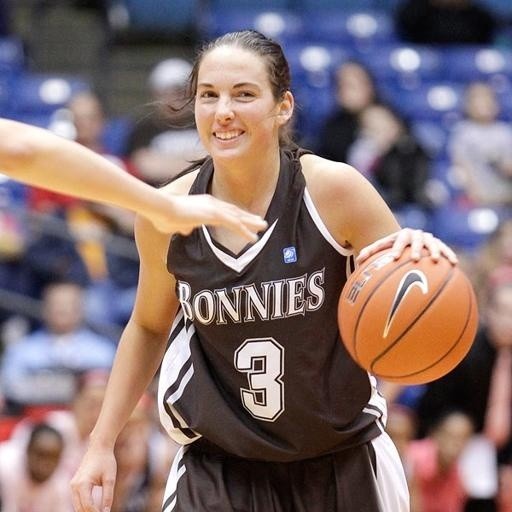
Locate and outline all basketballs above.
[338,244,478,387]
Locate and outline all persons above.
[65,27,464,512]
[1,54,510,512]
[0,113,270,247]
